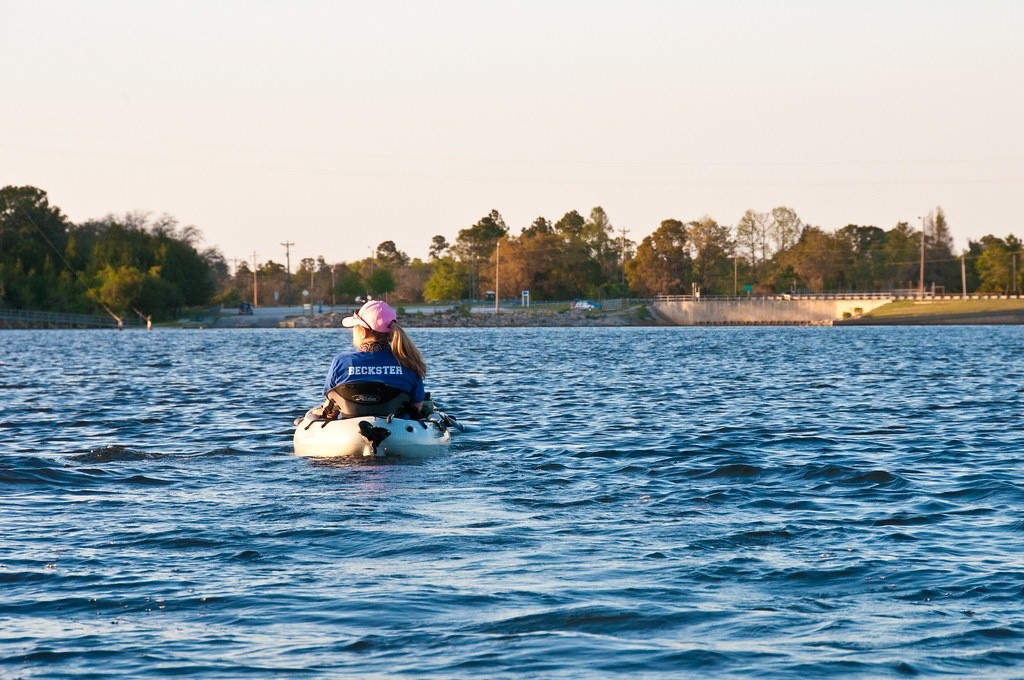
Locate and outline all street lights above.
[229,257,241,278]
[618,226,631,282]
[280,241,294,307]
[919,215,929,296]
[368,245,375,281]
[248,251,260,308]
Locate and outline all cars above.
[574,299,602,311]
[355,296,368,305]
[239,302,254,315]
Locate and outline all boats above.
[293,399,456,459]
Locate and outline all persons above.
[313,301,425,420]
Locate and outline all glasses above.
[353,309,377,336]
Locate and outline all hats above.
[342,300,398,332]
